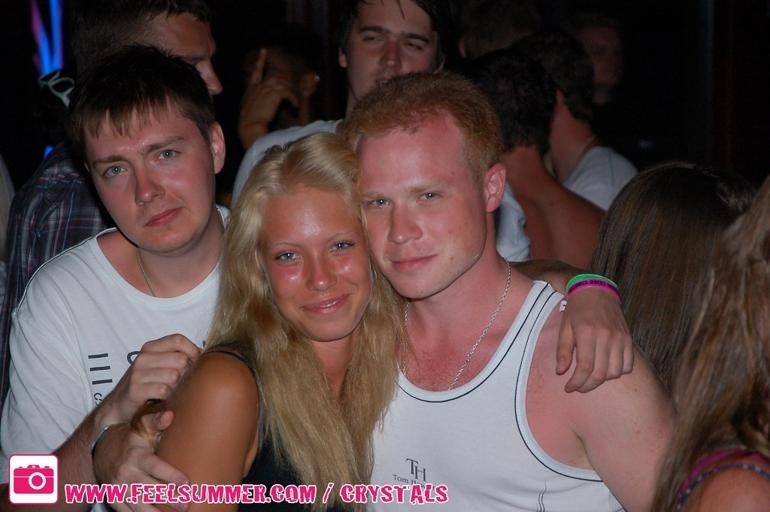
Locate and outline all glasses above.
[37,68,77,109]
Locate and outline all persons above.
[2,1,770,511]
